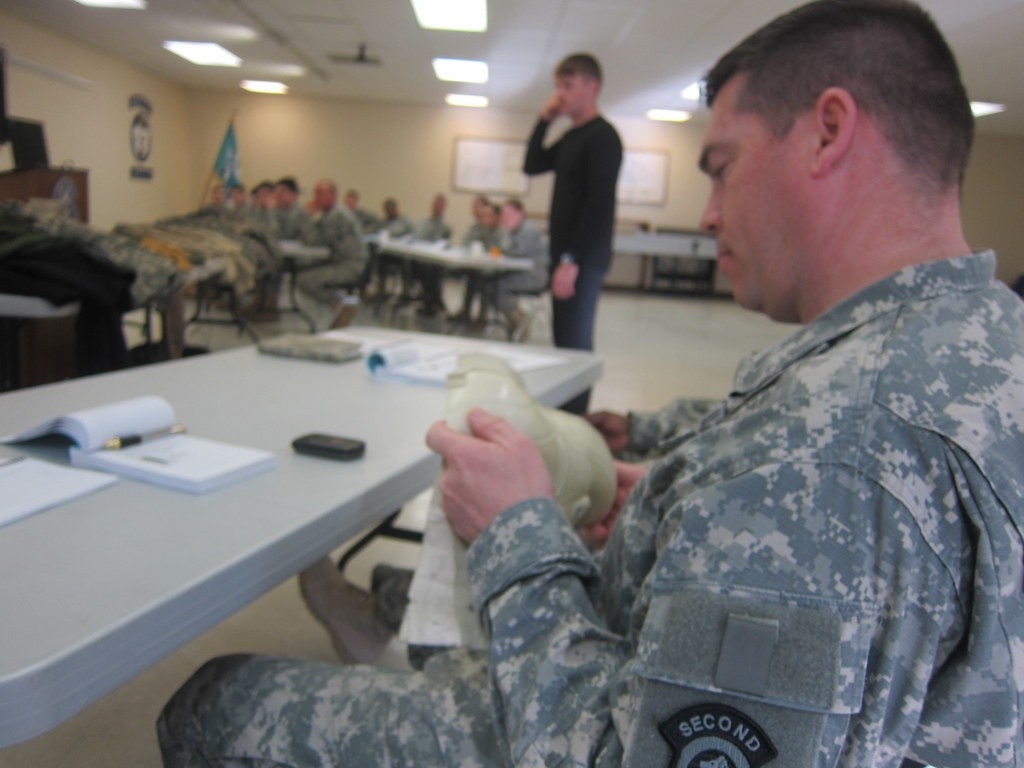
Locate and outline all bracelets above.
[560,255,574,263]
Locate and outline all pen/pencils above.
[94,423,187,451]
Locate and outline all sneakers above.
[299,556,393,665]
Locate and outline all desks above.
[135,233,533,346]
[614,233,720,296]
[0,324,605,750]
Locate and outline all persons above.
[523,53,622,417]
[195,176,549,342]
[155,0,1024,768]
[298,398,724,665]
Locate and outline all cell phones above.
[293,435,364,461]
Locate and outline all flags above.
[212,124,239,197]
